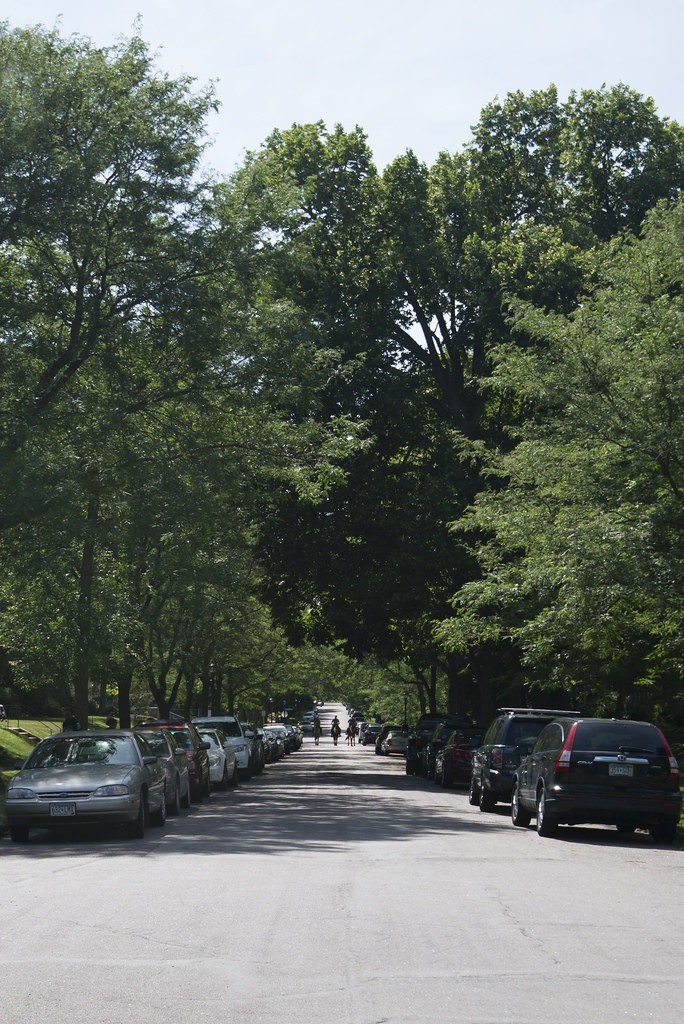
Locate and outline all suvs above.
[405,713,474,777]
[469,708,581,813]
[190,715,252,783]
[133,718,211,804]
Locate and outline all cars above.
[127,728,191,816]
[239,706,319,778]
[511,717,682,847]
[421,719,482,779]
[197,728,237,791]
[341,699,409,757]
[4,729,168,842]
[434,729,487,787]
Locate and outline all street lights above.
[400,690,410,729]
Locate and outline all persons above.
[401,721,408,732]
[106,718,117,729]
[313,716,323,737]
[63,709,82,733]
[346,715,357,735]
[330,715,342,737]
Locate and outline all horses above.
[313,721,361,747]
